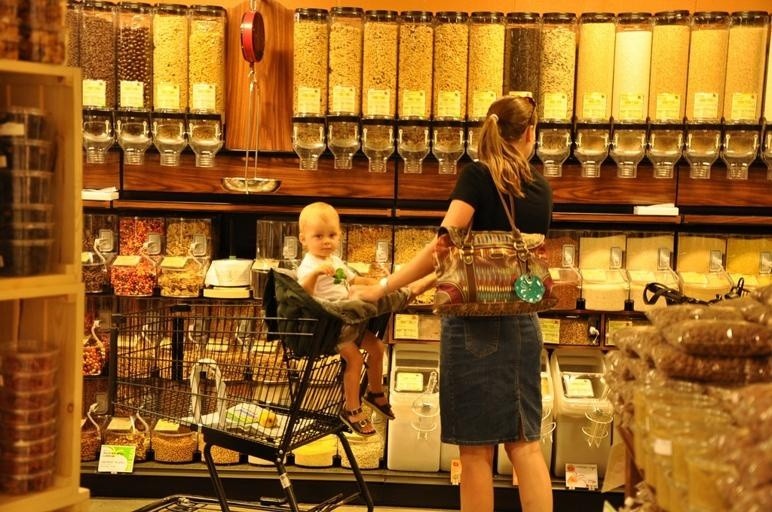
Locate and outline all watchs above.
[378,276,391,293]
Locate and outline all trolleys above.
[107,287,410,512]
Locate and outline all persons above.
[295,201,396,437]
[348,96,555,512]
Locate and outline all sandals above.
[361,387,396,421]
[338,402,377,438]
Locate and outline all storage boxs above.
[627,233,680,312]
[389,343,441,475]
[293,383,338,467]
[676,231,732,302]
[338,382,388,477]
[83,214,119,298]
[578,233,630,311]
[392,223,439,307]
[114,298,159,379]
[111,214,165,296]
[249,385,293,467]
[161,300,210,385]
[552,344,614,486]
[496,344,556,482]
[726,236,772,298]
[540,238,579,311]
[249,300,297,386]
[160,218,213,298]
[108,377,157,461]
[79,380,108,465]
[201,380,250,469]
[253,217,301,299]
[209,300,254,384]
[157,376,197,471]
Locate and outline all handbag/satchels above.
[431,159,560,318]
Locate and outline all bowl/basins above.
[219,177,282,193]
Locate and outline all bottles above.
[504,12,541,105]
[363,9,398,119]
[151,4,189,113]
[467,12,505,122]
[647,10,690,124]
[397,11,434,120]
[574,11,615,124]
[723,11,769,125]
[684,11,729,124]
[539,12,577,123]
[611,13,652,124]
[330,6,363,116]
[293,8,328,117]
[432,11,468,121]
[189,4,226,115]
[79,2,116,111]
[117,2,152,112]
